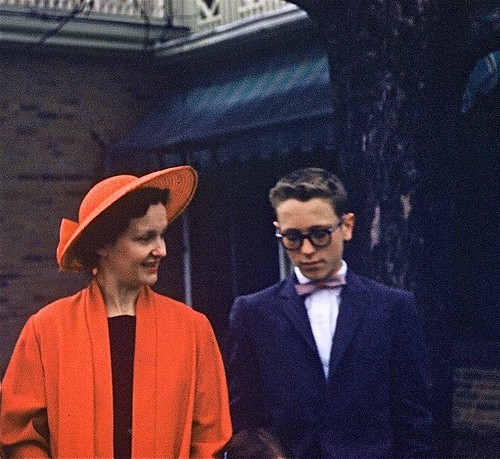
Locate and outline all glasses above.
[275,215,344,251]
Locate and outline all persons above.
[0,166,233,459]
[222,167,441,459]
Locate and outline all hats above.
[57,166,197,271]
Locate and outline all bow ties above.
[295,275,347,296]
